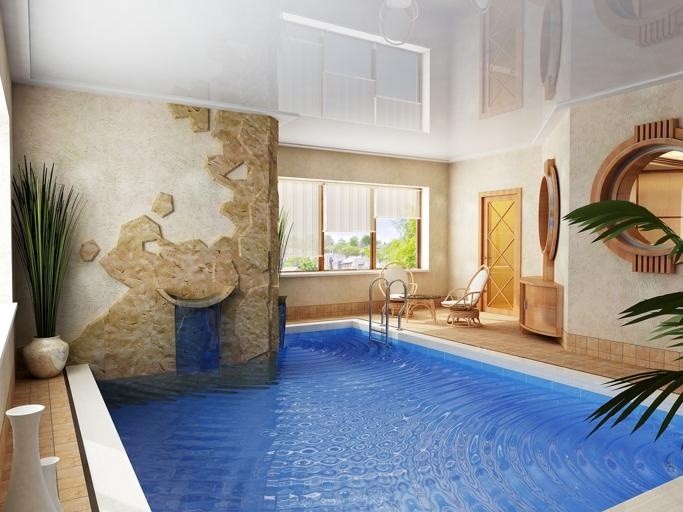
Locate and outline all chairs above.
[441,264,489,329]
[379,262,418,317]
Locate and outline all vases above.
[42,457,62,511]
[5,405,54,512]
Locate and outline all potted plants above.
[12,154,87,380]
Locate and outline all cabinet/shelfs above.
[520,277,564,339]
